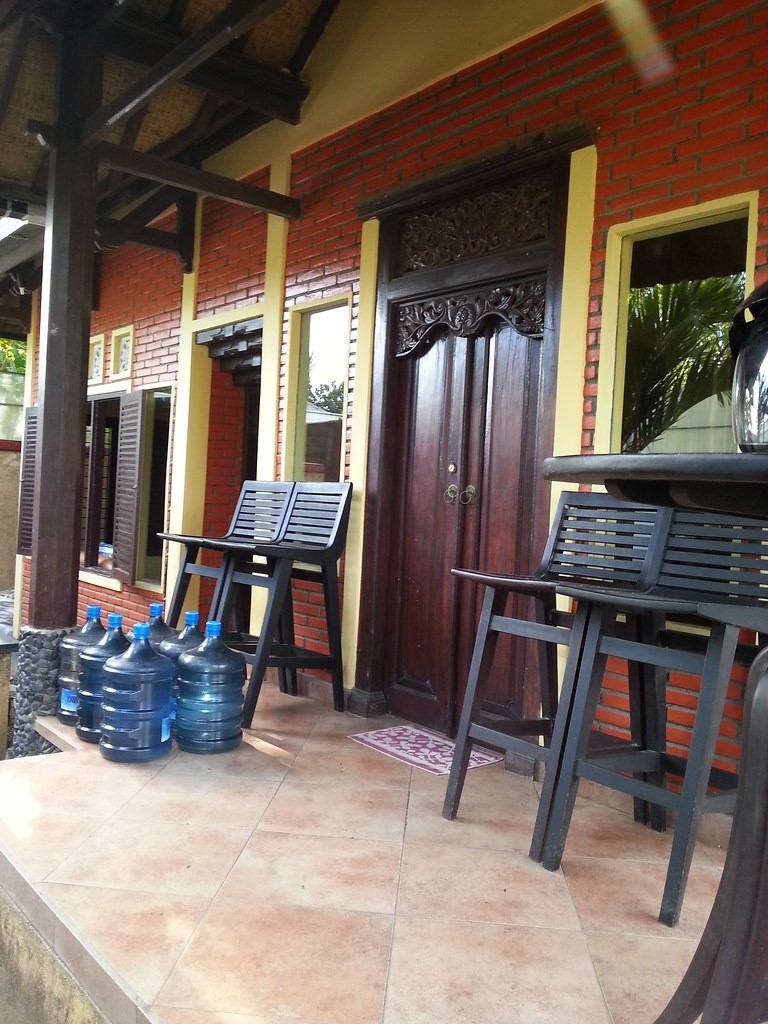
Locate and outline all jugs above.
[728,279,768,453]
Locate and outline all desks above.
[542,453,768,1024]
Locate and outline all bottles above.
[56,606,107,727]
[159,612,206,739]
[127,604,181,651]
[75,614,131,745]
[98,623,175,763]
[176,621,245,755]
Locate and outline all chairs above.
[203,482,352,729]
[544,505,768,929]
[157,480,295,692]
[441,490,671,863]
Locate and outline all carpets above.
[348,724,503,776]
